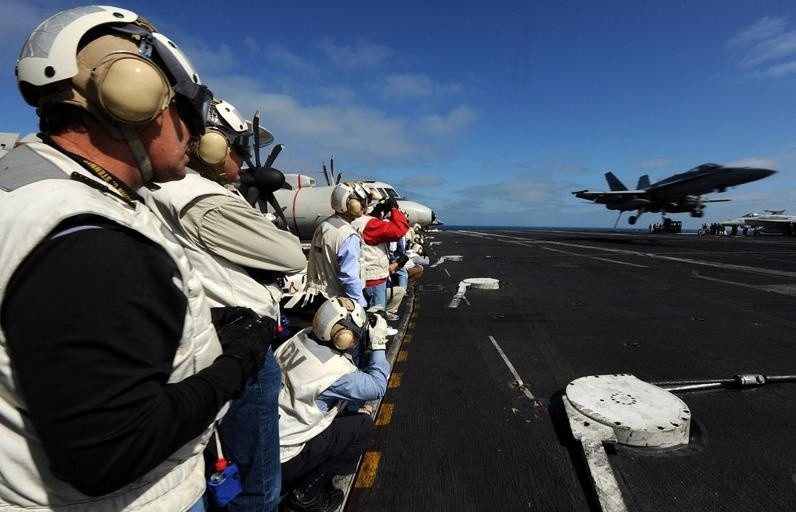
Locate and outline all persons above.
[1,2,280,511]
[148,96,308,511]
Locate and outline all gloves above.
[210,304,280,379]
[368,314,389,352]
[386,195,400,209]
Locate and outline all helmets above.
[204,97,250,149]
[13,6,212,137]
[312,292,367,351]
[330,179,382,217]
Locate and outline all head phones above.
[348,199,360,214]
[335,329,353,351]
[195,133,228,163]
[90,54,171,123]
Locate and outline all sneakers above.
[283,486,346,512]
[387,309,400,338]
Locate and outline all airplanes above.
[233,111,436,246]
[569,159,796,235]
